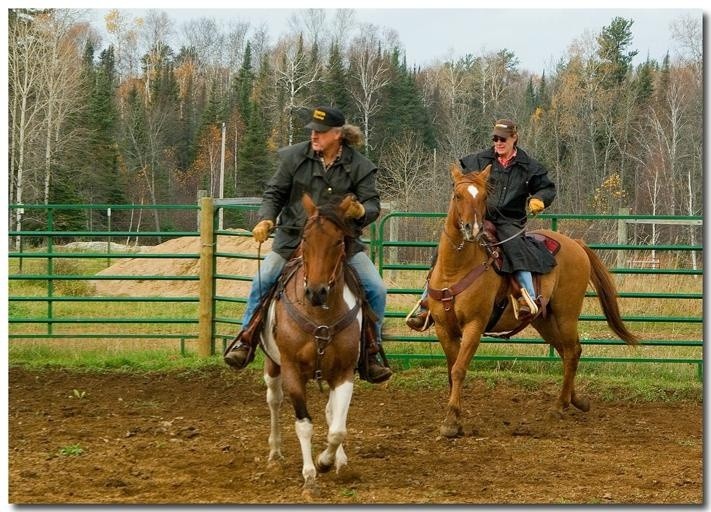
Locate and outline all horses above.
[257,191,366,499]
[427,162,644,437]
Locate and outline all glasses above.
[492,137,508,142]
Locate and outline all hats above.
[303,105,346,133]
[489,118,518,138]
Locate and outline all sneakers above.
[358,354,393,383]
[222,345,256,369]
[406,311,434,331]
[516,296,544,319]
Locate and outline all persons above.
[224,107,393,383]
[406,119,558,327]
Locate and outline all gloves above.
[251,218,275,243]
[527,198,545,213]
[340,194,366,221]
